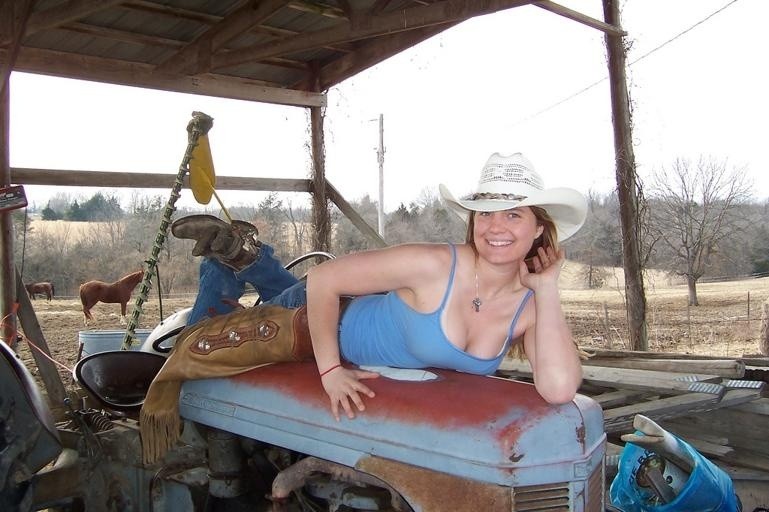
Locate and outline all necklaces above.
[472,258,516,313]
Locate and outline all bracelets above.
[319,364,342,377]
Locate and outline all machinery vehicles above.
[0,251,607,510]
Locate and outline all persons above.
[171,151,584,423]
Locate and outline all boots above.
[172,215,258,271]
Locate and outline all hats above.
[439,153,587,243]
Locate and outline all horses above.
[79,271,144,327]
[25,282,55,301]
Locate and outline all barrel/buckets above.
[79,328,172,355]
